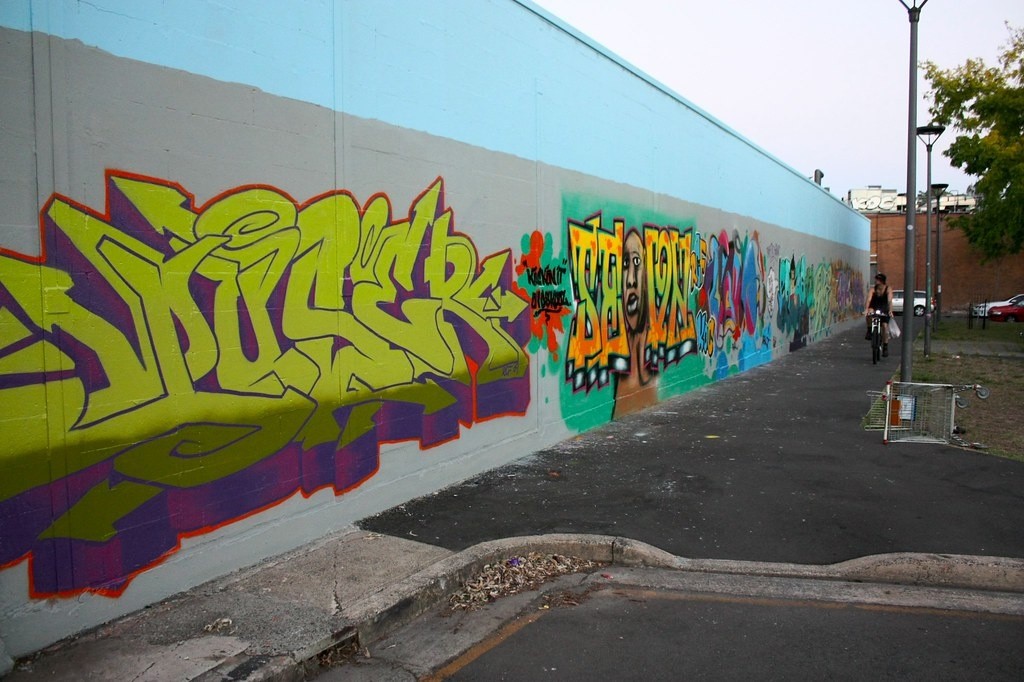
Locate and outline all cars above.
[972,293,1024,323]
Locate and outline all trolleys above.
[863,378,989,450]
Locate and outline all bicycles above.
[861,310,890,365]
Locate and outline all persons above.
[864,274,893,358]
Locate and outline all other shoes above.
[882,346,888,357]
[865,326,872,340]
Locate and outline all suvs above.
[891,290,936,318]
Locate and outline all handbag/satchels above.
[887,317,901,339]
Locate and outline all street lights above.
[930,184,949,319]
[916,123,946,357]
[896,0,929,391]
